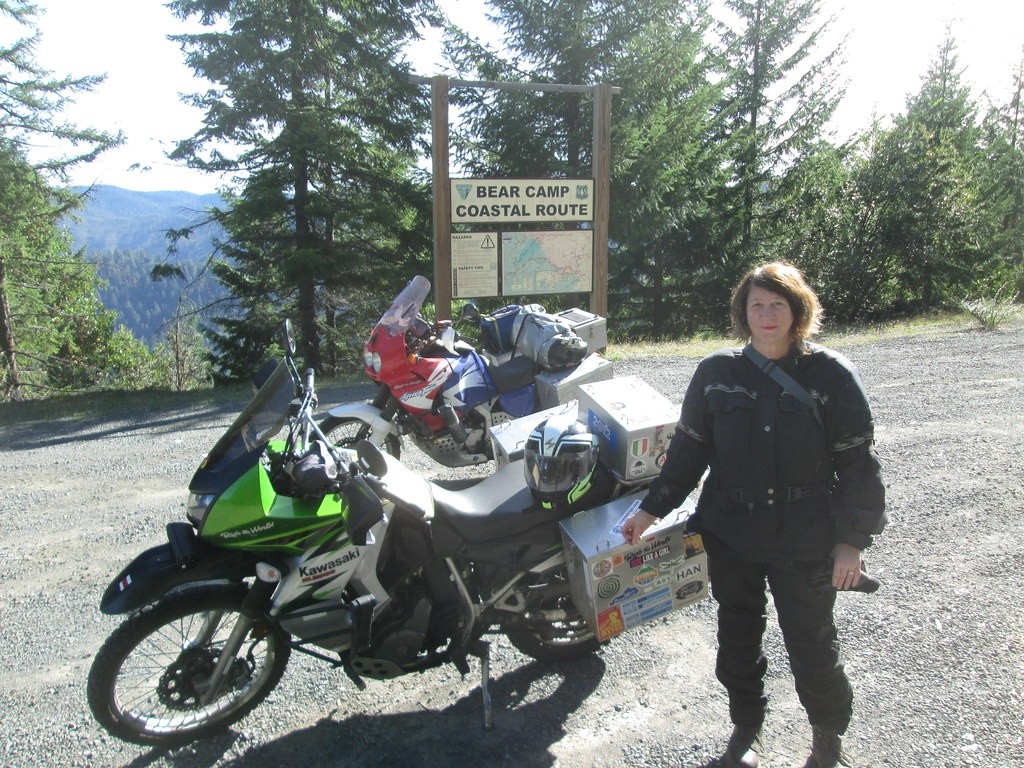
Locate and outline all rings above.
[848,571,856,575]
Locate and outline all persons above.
[617,262,887,768]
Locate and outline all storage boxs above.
[481,349,540,394]
[535,353,613,411]
[577,374,682,480]
[558,489,707,641]
[555,307,608,352]
[488,400,578,472]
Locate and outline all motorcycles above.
[306,275,615,469]
[84,317,711,748]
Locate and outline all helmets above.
[524,416,604,512]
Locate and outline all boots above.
[806,724,842,768]
[726,724,760,768]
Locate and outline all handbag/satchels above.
[511,303,588,368]
